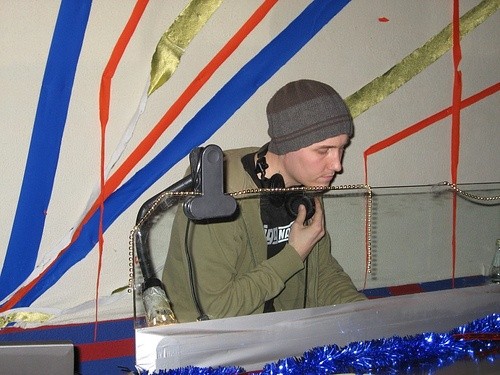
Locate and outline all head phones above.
[256,142,316,221]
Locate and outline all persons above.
[162,79,369,323]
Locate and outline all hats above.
[266,79,354,155]
